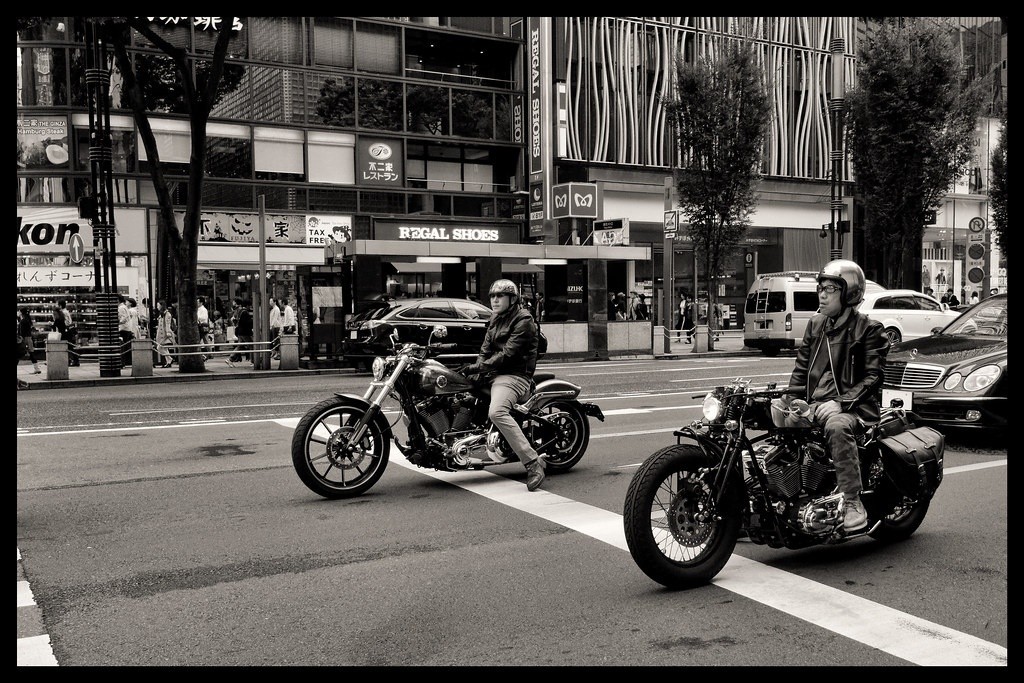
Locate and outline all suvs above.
[342,297,548,374]
[856,288,980,342]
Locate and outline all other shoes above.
[225,358,234,369]
[250,362,254,367]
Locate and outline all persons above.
[607,292,724,344]
[770,259,889,532]
[927,287,999,309]
[923,266,946,290]
[119,292,296,368]
[17,307,41,374]
[43,300,80,367]
[457,279,546,490]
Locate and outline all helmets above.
[816,259,866,306]
[489,279,520,299]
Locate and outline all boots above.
[29,362,41,374]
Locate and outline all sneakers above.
[526,457,546,491]
[843,495,867,531]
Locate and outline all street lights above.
[820,224,835,261]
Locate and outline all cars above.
[885,292,1009,441]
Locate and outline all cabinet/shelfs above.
[17,292,99,350]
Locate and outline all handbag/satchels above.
[163,311,176,331]
[202,326,210,334]
[235,326,239,338]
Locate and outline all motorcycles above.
[291,323,604,501]
[622,376,949,589]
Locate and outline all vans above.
[742,271,887,356]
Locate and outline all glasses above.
[817,284,842,294]
[490,293,516,298]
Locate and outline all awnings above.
[392,264,544,273]
